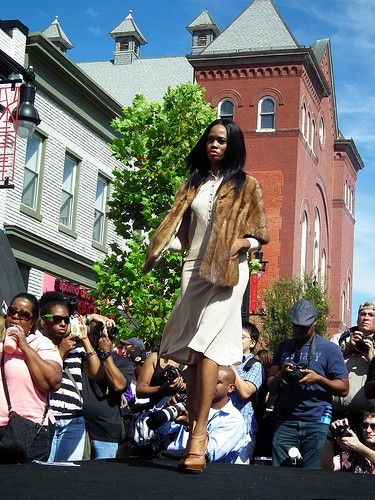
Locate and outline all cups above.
[4,326,21,354]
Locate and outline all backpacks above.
[243,358,267,427]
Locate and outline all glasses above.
[360,422,375,431]
[90,329,101,337]
[242,334,251,341]
[7,307,33,322]
[122,344,132,350]
[41,313,70,325]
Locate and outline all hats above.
[290,301,318,325]
[119,337,145,350]
[133,350,152,365]
[358,302,375,313]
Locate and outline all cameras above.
[333,424,351,438]
[145,395,188,431]
[162,365,177,384]
[355,337,371,350]
[92,325,119,340]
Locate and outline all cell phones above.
[286,364,311,381]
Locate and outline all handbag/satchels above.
[0,411,43,461]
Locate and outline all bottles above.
[288,447,303,465]
[321,405,332,425]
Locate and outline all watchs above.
[101,352,111,359]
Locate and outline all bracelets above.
[87,351,96,357]
[86,314,89,325]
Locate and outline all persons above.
[267,300,349,469]
[0,292,63,462]
[39,290,103,461]
[253,339,295,463]
[331,301,375,456]
[331,411,375,474]
[229,322,265,464]
[119,338,189,457]
[142,118,270,470]
[168,365,246,463]
[66,313,132,459]
